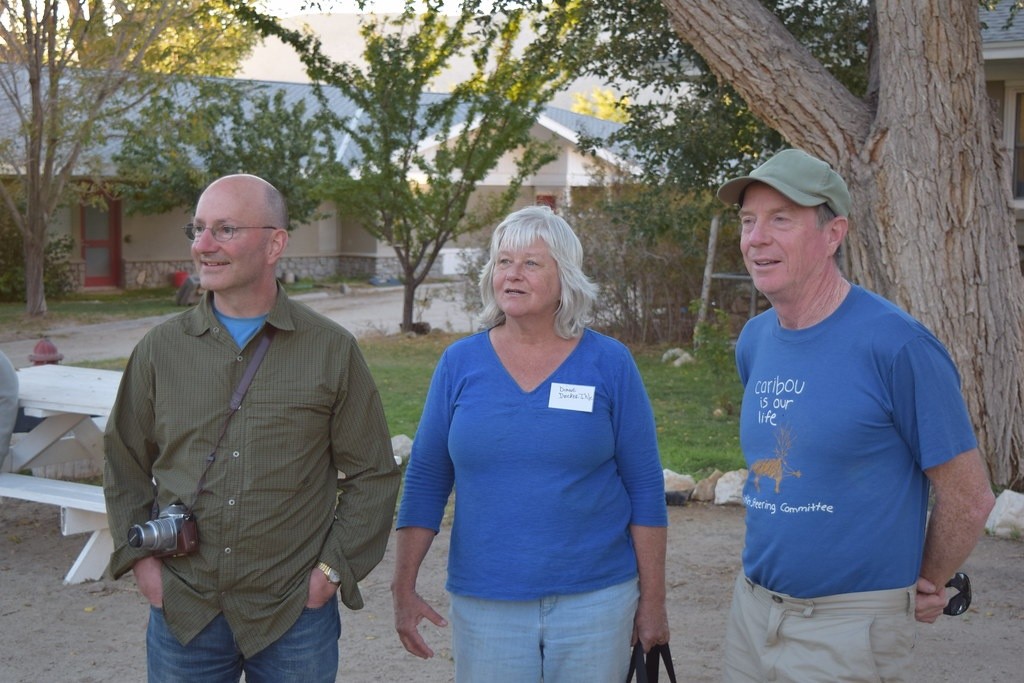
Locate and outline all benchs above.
[0,416,403,587]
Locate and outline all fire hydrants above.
[29,339,65,366]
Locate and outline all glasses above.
[941,572,971,616]
[181,222,278,243]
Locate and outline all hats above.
[716,149,851,217]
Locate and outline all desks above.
[15,363,124,587]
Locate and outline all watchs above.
[314,561,341,585]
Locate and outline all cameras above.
[127,503,200,558]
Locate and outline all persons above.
[717,148,996,683]
[0,350,18,472]
[103,173,402,683]
[390,205,670,683]
